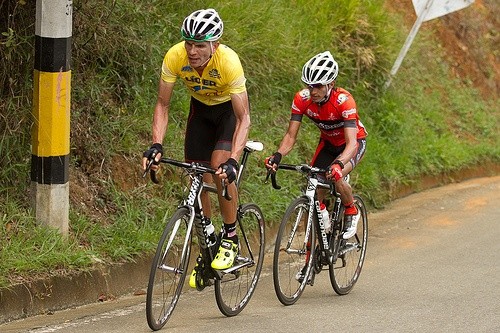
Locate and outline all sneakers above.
[343,206,361,240]
[188,253,207,289]
[295,265,308,281]
[210,238,240,270]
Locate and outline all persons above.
[265,51,368,286]
[144,9,250,288]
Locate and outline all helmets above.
[300,52,339,86]
[180,8,224,42]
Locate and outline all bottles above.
[203,216,216,246]
[320,202,332,233]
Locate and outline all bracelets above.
[333,161,345,169]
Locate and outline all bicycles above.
[141,141,266,331]
[264,159,368,306]
